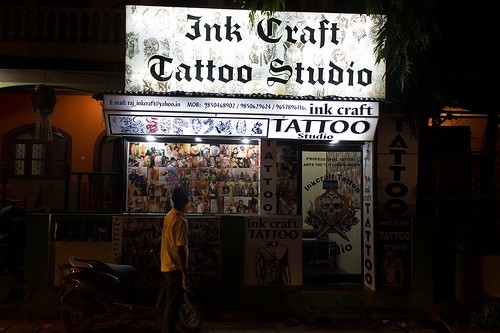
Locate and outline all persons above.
[128,142,259,214]
[160,187,191,333]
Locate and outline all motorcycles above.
[57,249,202,333]
